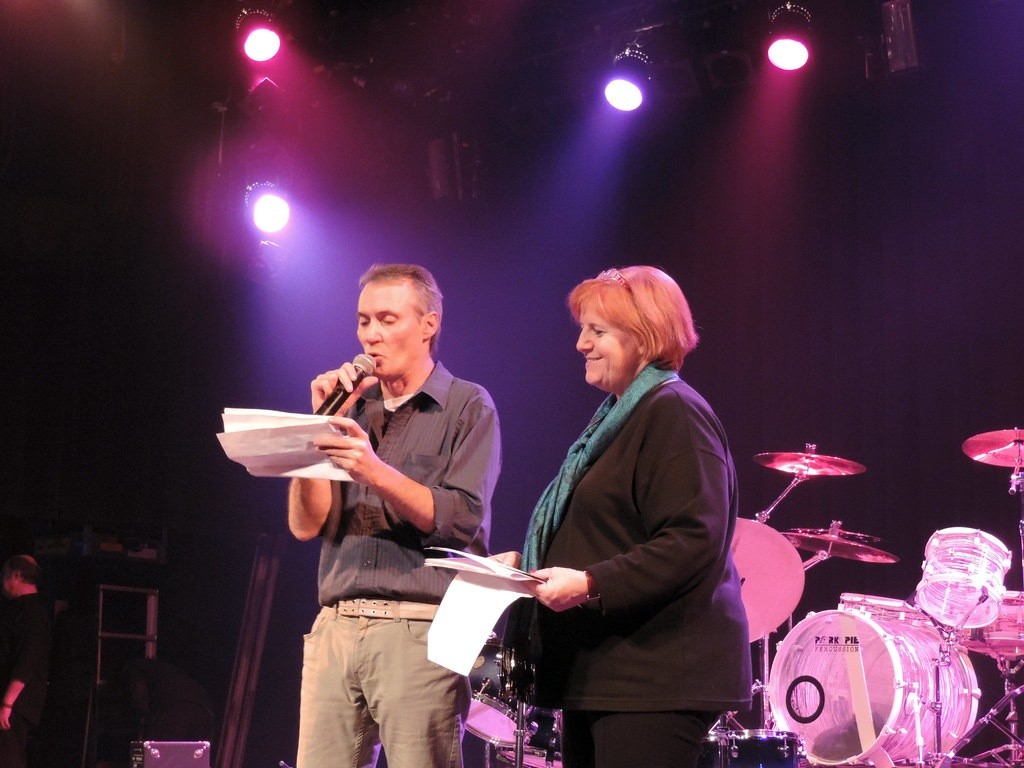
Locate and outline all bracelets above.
[0,703,12,709]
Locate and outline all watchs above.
[584,571,600,611]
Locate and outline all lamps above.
[602,7,657,114]
[230,0,281,63]
[767,0,815,72]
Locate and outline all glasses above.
[595,269,631,292]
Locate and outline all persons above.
[487,265,755,768]
[0,555,50,768]
[289,263,502,767]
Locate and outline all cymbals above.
[752,452,867,477]
[962,429,1024,465]
[779,531,900,563]
[790,528,881,543]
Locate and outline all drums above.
[958,590,1024,657]
[494,707,563,768]
[837,592,905,615]
[464,639,537,745]
[768,609,981,766]
[915,527,1012,629]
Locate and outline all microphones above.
[314,354,376,416]
